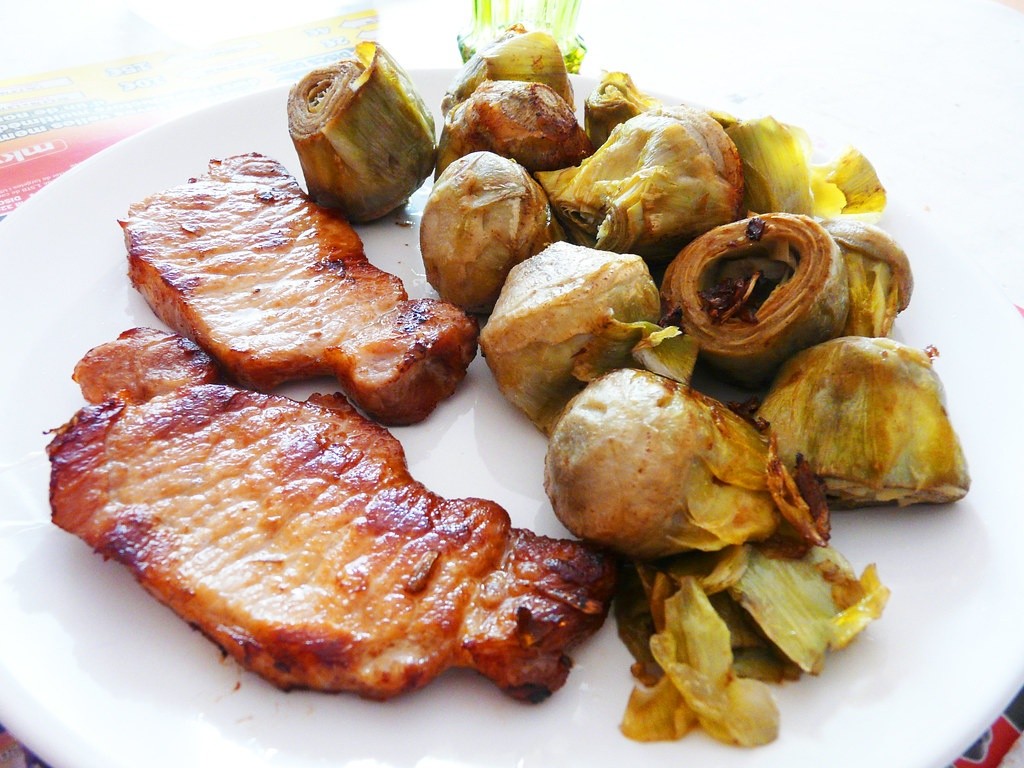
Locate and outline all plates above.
[0,65,1024,768]
